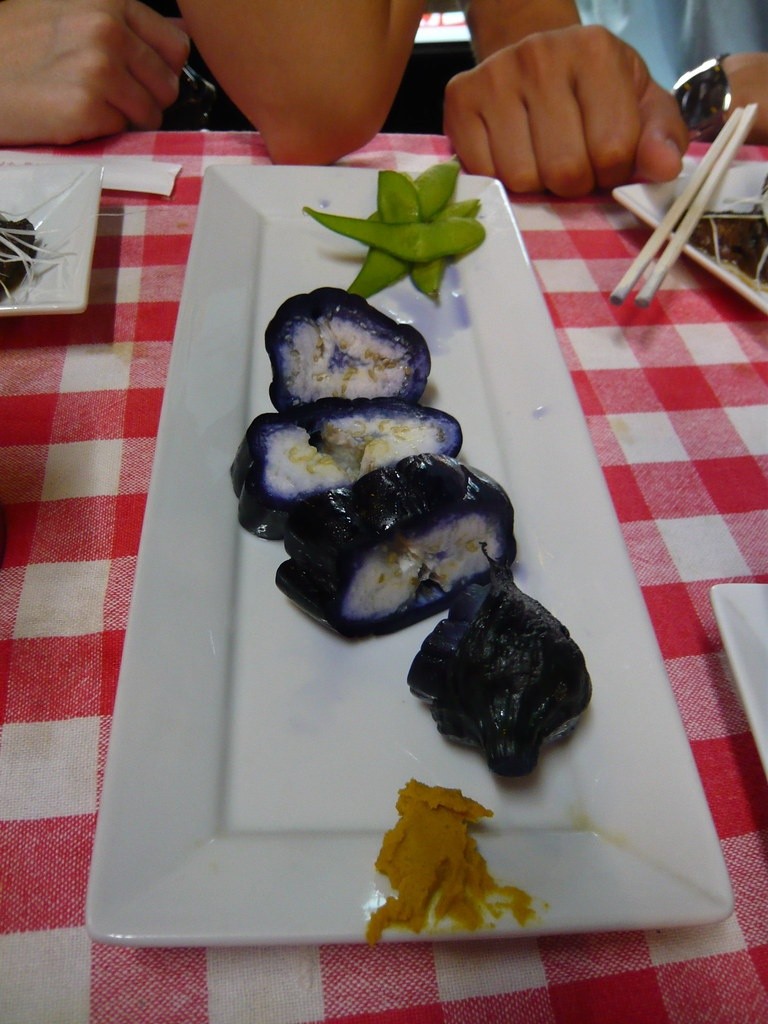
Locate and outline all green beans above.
[303,163,487,301]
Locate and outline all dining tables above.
[0,127,767,1024]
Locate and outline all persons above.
[443,0,768,199]
[0,0,425,165]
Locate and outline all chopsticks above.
[609,103,759,308]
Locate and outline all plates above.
[613,160,768,316]
[86,164,734,947]
[709,584,767,777]
[1,161,106,316]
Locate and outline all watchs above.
[673,52,732,143]
